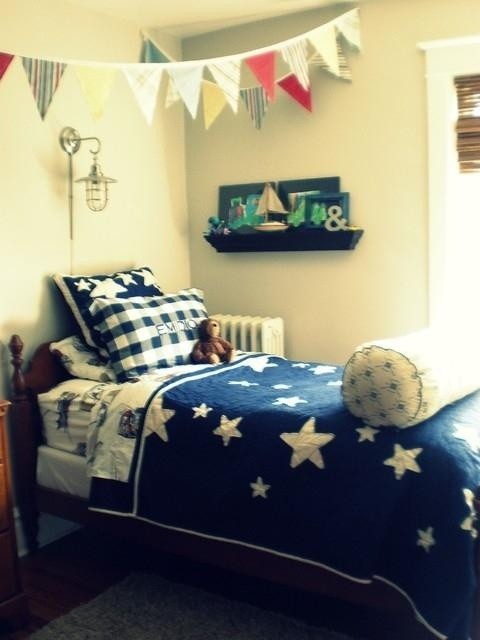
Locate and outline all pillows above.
[51,268,212,385]
[339,316,480,427]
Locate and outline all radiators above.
[207,312,286,357]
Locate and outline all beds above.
[9,329,480,635]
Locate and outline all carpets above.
[14,566,353,639]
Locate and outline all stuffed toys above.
[191,318,237,364]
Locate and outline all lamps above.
[60,121,115,210]
